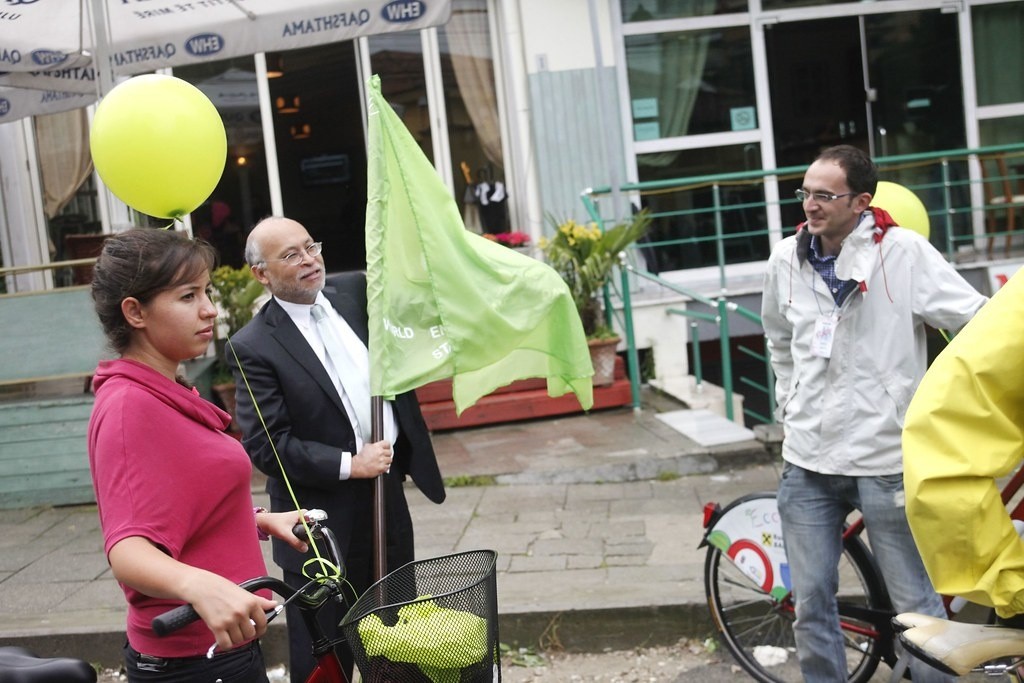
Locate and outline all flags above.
[364,74,595,418]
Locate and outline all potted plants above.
[560,337,622,388]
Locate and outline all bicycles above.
[696,489,1024,683]
[0,510,505,683]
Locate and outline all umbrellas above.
[0,0,453,243]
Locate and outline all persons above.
[88,227,314,683]
[761,145,1024,683]
[225,216,445,683]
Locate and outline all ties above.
[311,304,373,444]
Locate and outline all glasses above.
[258,241,323,266]
[794,188,859,203]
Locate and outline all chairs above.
[978,150,1024,257]
[66,233,120,392]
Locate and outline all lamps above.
[266,53,285,78]
[277,92,301,113]
[287,118,313,141]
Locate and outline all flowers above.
[536,207,653,341]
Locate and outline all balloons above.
[869,181,930,241]
[89,72,228,220]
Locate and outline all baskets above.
[337,550,501,683]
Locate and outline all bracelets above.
[253,506,269,541]
